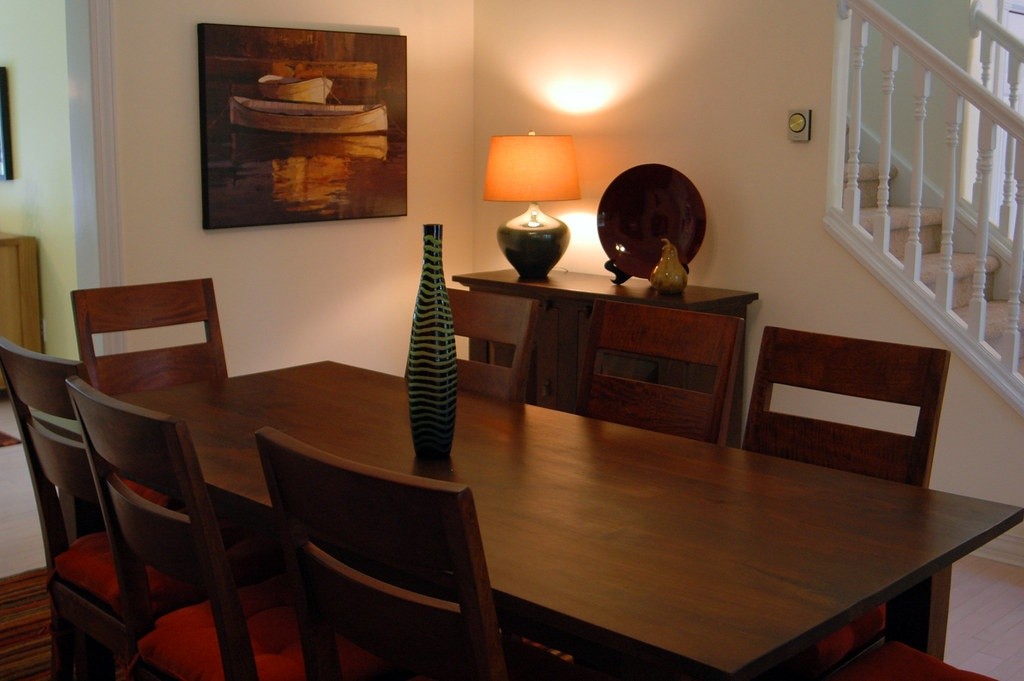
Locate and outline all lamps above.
[484,128,583,280]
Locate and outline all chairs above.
[743,326,955,669]
[452,287,540,402]
[60,376,401,681]
[575,296,746,442]
[65,274,230,531]
[249,427,625,681]
[0,339,281,681]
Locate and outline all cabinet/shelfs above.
[0,229,44,396]
[448,267,759,445]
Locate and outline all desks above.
[99,358,1024,681]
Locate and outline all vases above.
[405,220,456,458]
